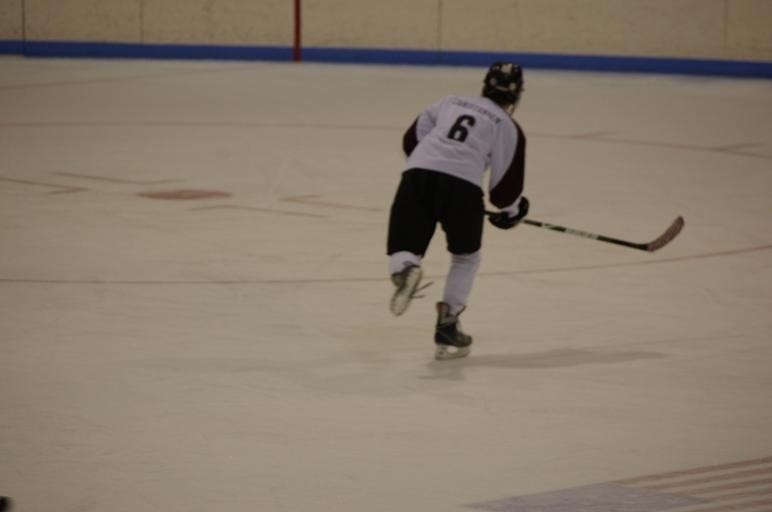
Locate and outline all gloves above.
[487,197,529,230]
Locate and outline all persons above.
[385,60,529,346]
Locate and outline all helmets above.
[484,62,523,94]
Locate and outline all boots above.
[434,301,472,347]
[390,264,421,317]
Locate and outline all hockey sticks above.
[484,210,685,252]
